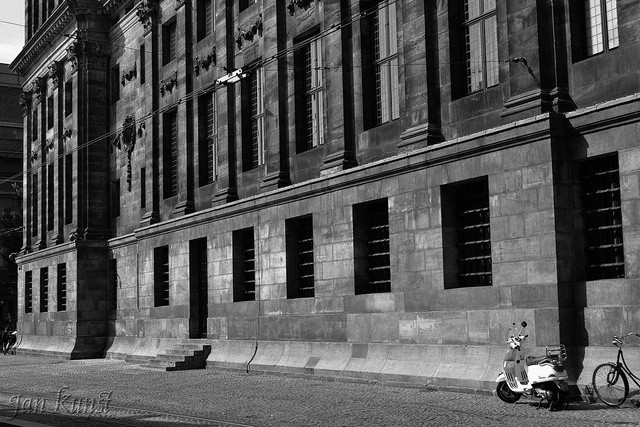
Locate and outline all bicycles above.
[592,332,639,408]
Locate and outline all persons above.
[1,327,17,355]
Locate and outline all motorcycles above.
[495,320,570,411]
